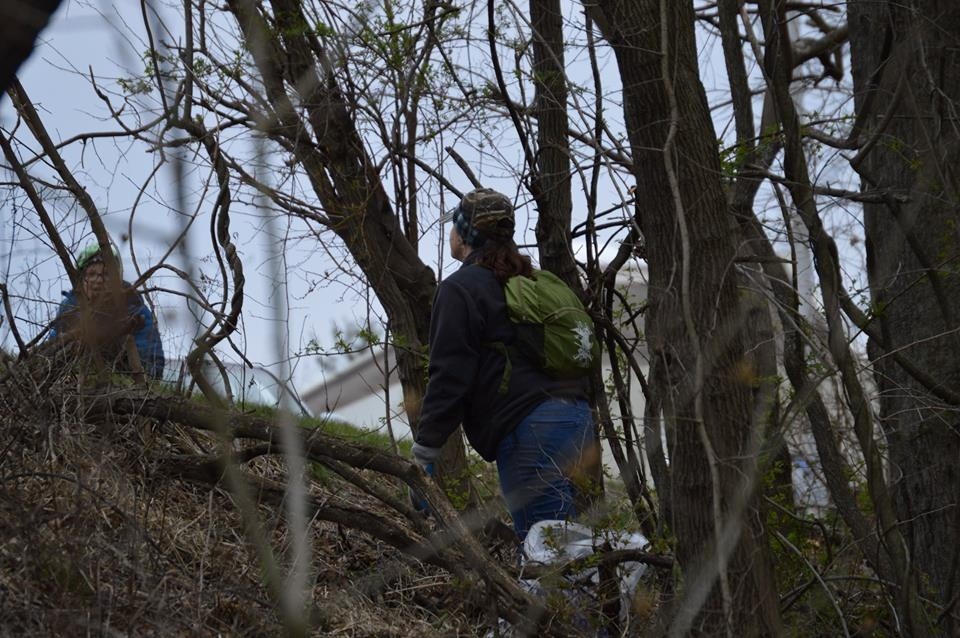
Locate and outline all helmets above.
[77,243,122,278]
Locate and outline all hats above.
[452,188,515,244]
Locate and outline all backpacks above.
[494,265,600,377]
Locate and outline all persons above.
[407,186,595,638]
[39,249,165,385]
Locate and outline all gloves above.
[410,461,434,520]
